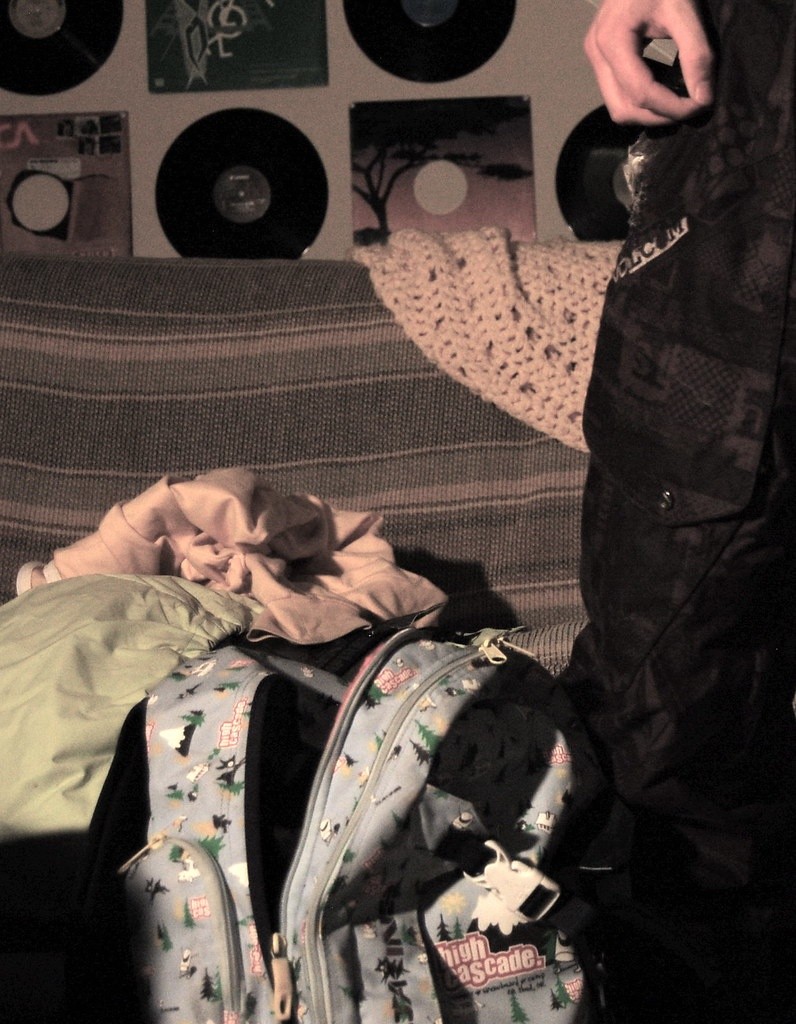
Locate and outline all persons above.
[546,0,796,1024]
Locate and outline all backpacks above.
[121,628,617,1024]
